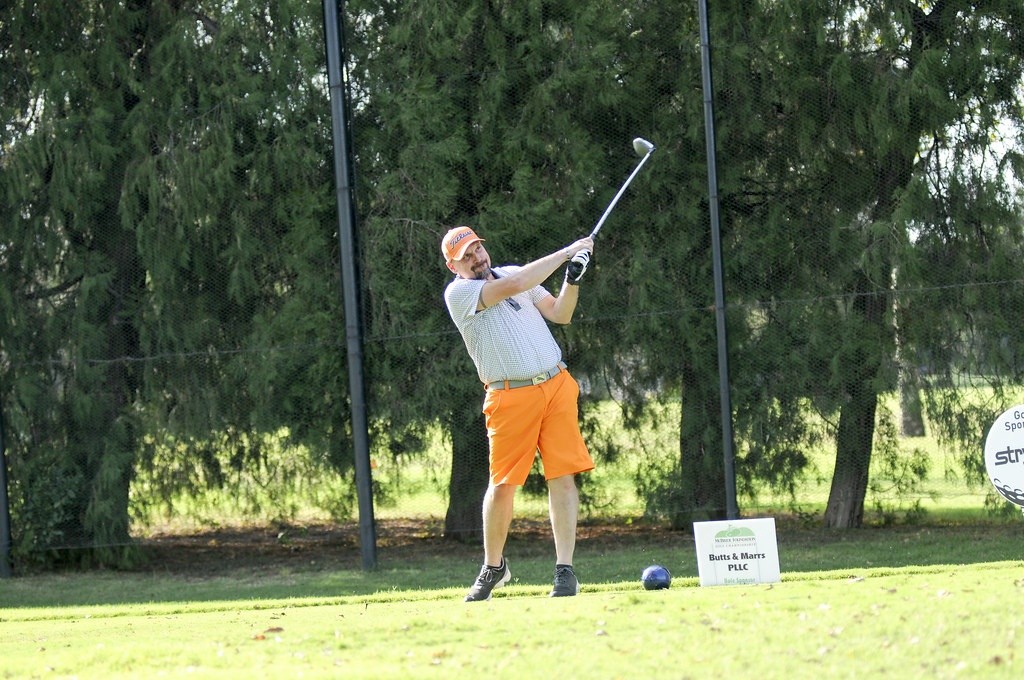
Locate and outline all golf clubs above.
[566,137,657,285]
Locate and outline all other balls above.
[641,564,672,590]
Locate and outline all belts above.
[486,358,568,390]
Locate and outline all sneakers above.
[549,567,580,597]
[463,554,511,602]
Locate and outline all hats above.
[441,225,486,263]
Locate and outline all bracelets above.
[565,248,571,260]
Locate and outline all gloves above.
[565,248,591,286]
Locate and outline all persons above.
[441,227,594,601]
